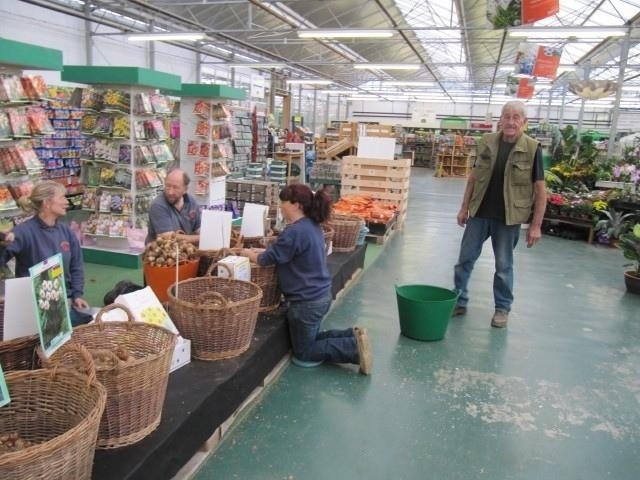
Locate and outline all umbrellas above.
[568,66,619,99]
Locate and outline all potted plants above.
[541,118,640,245]
[620,222,640,295]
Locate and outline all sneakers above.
[490,308,509,328]
[452,303,468,314]
[352,326,372,375]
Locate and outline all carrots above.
[331,195,397,225]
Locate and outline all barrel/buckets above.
[394,284,463,342]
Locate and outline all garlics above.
[39,279,63,311]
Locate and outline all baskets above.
[167,208,283,362]
[321,213,362,257]
[0,296,177,480]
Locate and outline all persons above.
[452,101,546,328]
[238,184,370,375]
[0,181,93,328]
[104,168,203,308]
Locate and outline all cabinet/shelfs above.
[182,81,248,217]
[62,64,182,269]
[339,153,410,233]
[231,100,342,215]
[339,99,489,177]
[0,38,65,245]
